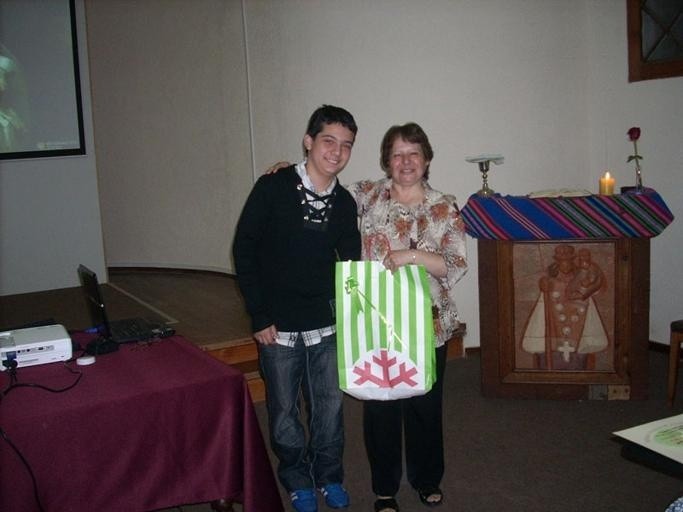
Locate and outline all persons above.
[226,103,363,512]
[266,121,471,510]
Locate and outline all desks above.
[0,330,288,512]
[454,187,674,402]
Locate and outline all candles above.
[598,172,618,197]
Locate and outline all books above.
[528,187,592,198]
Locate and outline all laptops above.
[77,264,176,344]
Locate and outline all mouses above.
[86,337,119,356]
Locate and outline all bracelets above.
[409,247,417,263]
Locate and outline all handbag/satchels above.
[333,259,438,401]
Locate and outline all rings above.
[387,262,393,266]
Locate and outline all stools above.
[662,318,682,410]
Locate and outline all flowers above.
[622,127,647,189]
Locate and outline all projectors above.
[0,323,73,371]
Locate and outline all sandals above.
[375,494,399,512]
[417,486,445,508]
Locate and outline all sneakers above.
[286,487,320,512]
[317,484,351,509]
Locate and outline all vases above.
[633,166,646,195]
